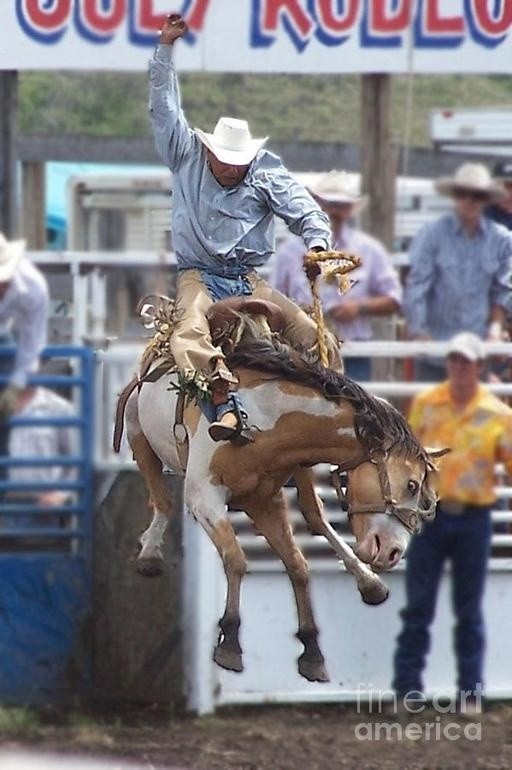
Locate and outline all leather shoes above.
[209,410,254,447]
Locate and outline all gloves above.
[1,384,22,418]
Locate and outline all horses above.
[124,312,451,683]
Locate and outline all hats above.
[444,330,491,362]
[307,169,370,206]
[434,162,509,207]
[0,230,28,284]
[191,116,271,167]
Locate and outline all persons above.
[144,6,335,448]
[266,162,407,387]
[0,223,52,548]
[485,158,512,236]
[387,329,512,729]
[399,161,512,393]
